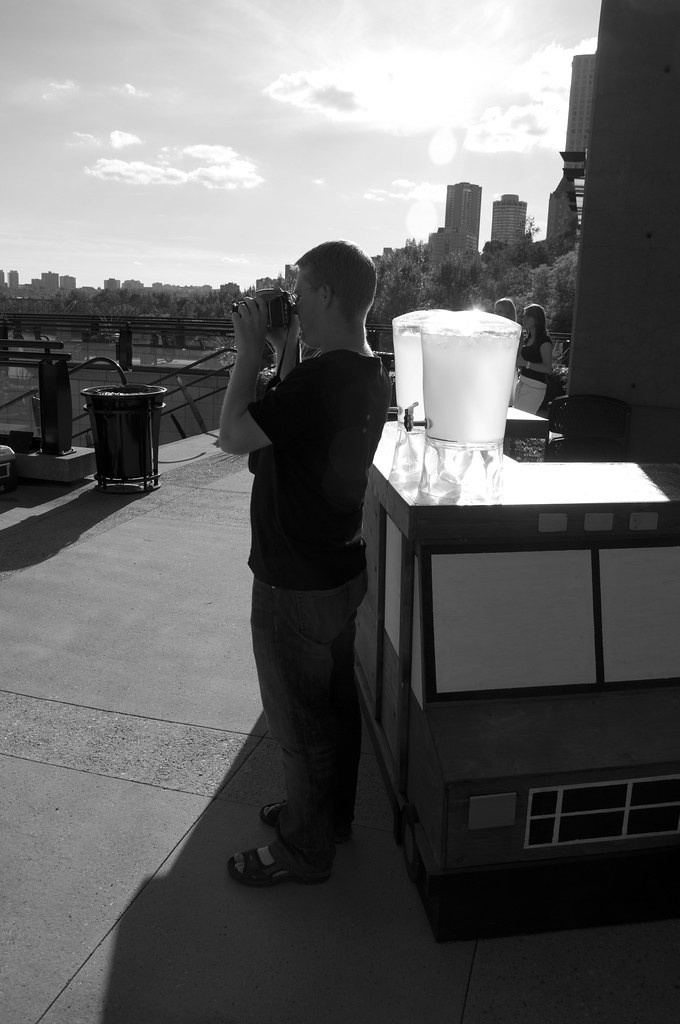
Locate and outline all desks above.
[506,407,550,462]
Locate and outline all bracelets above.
[526,362,530,369]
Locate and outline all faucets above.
[402,403,428,433]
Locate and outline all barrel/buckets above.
[392,309,453,431]
[404,312,522,449]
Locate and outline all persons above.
[494,299,553,416]
[216,242,392,889]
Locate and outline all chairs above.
[542,394,632,461]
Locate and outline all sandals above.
[260,799,353,844]
[226,839,333,886]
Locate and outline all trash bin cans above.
[79,382,170,494]
